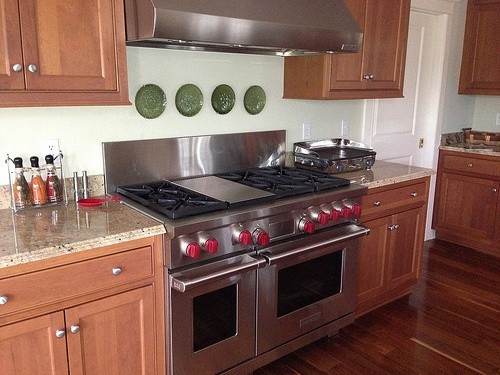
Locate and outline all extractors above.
[124,0,364,56]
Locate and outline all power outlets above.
[46,140,60,168]
[342,120,350,136]
[302,123,311,140]
[496,113,500,126]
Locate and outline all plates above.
[175,84,204,117]
[135,83,167,119]
[211,84,235,115]
[244,85,266,115]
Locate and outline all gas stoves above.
[114,164,368,238]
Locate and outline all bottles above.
[46,155,63,204]
[30,156,49,206]
[12,157,31,211]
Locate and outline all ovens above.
[168,194,363,375]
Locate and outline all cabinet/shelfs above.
[282,0,412,101]
[0,0,132,106]
[431,148,499,261]
[0,236,170,375]
[354,177,431,317]
[457,0,500,96]
